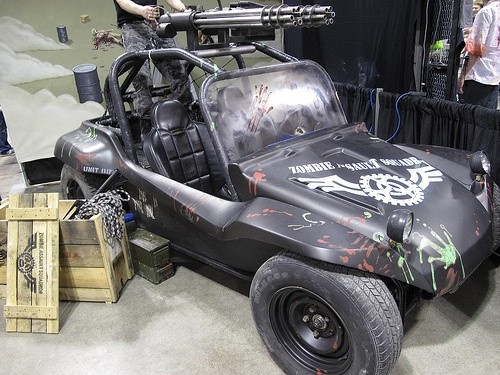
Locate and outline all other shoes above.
[4,148,15,156]
[189,108,205,122]
[140,118,152,135]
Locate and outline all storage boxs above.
[0,196,134,304]
[127,227,175,285]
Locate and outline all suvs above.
[53,40,499,372]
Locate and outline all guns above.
[62,169,119,220]
[152,4,336,51]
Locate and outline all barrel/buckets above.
[72,64,103,104]
[56,24,68,43]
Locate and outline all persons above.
[458,0,500,111]
[112,0,205,143]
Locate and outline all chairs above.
[213,86,278,162]
[142,99,232,203]
